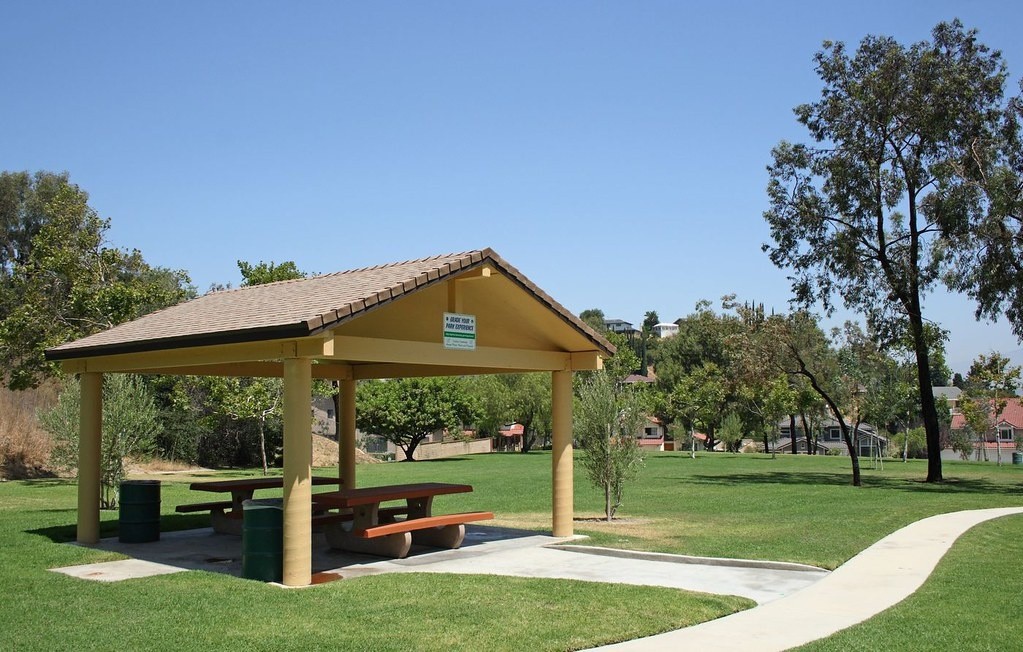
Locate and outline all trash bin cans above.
[242,498,283,584]
[118,480,161,543]
[1013,453,1023,464]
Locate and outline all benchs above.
[175,501,231,512]
[311,506,409,526]
[352,511,493,540]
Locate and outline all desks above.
[190,476,344,536]
[311,483,473,558]
